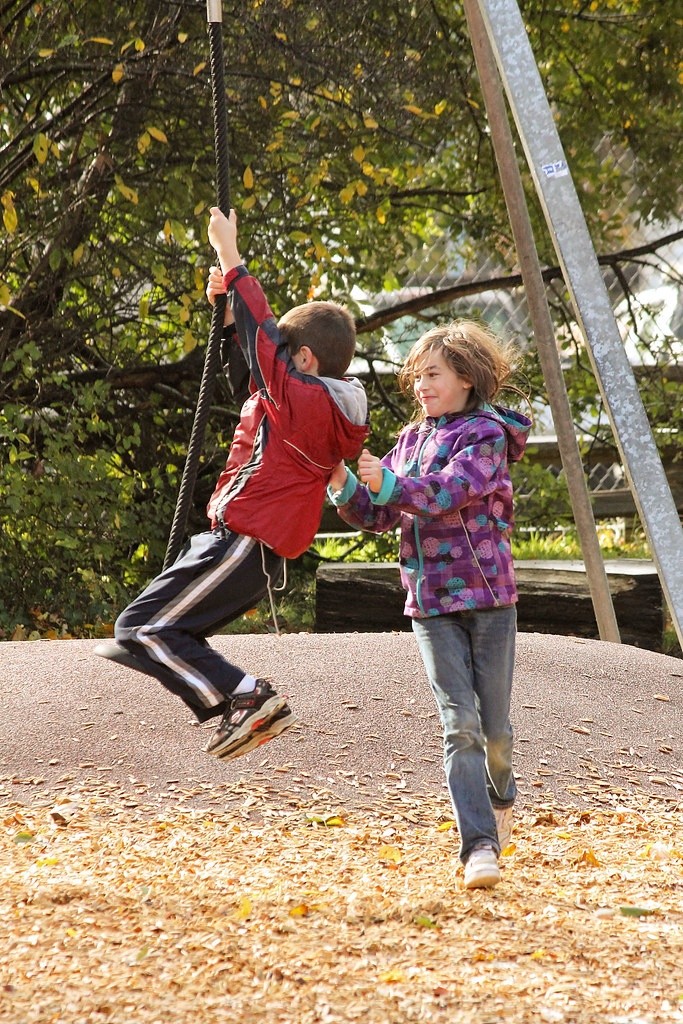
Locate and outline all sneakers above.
[493,807,512,852]
[205,678,285,755]
[217,694,299,761]
[464,841,500,888]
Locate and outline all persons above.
[328,323,532,887]
[114,208,371,760]
